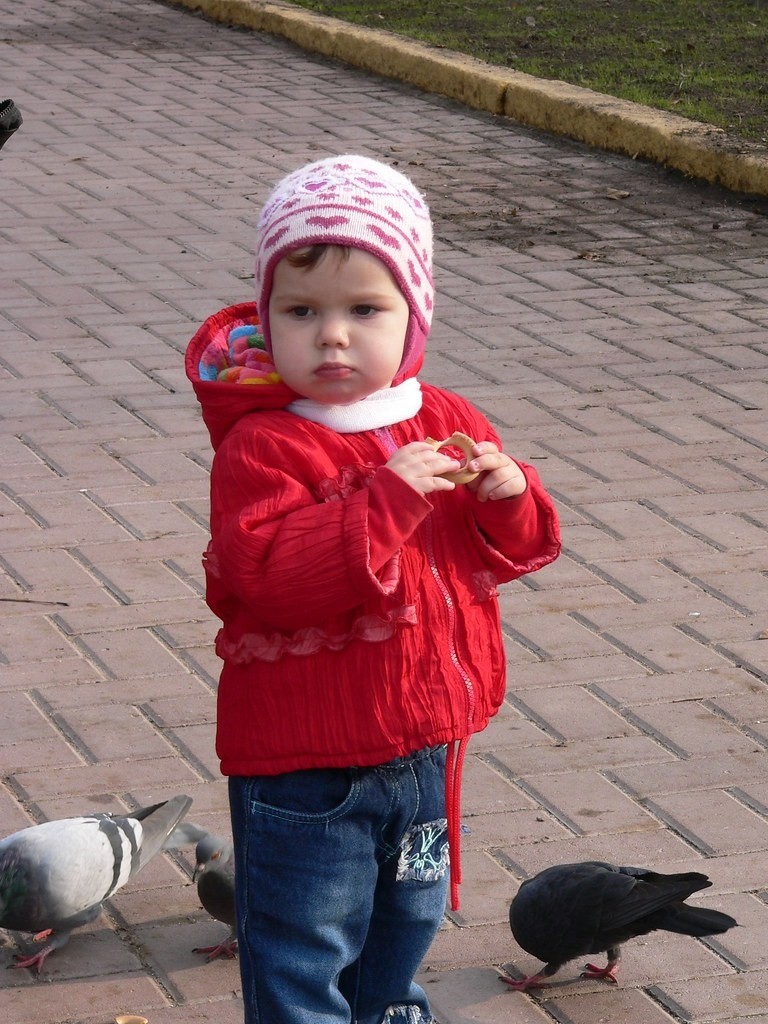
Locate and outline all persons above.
[184,155,563,1024]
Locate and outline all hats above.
[255,155,434,381]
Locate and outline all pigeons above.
[190,836,238,964]
[0,794,194,976]
[497,862,746,992]
